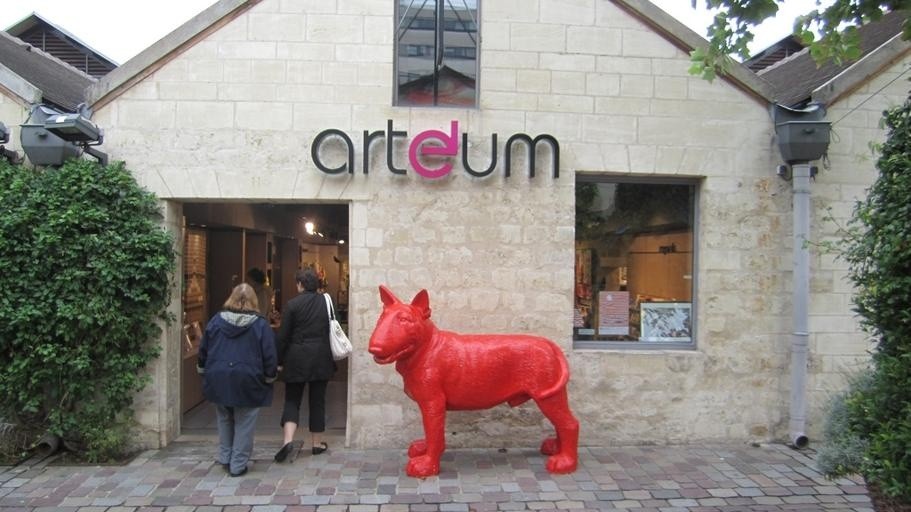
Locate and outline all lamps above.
[43,112,108,167]
[18,124,79,167]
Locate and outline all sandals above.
[275,441,293,462]
[312,442,328,454]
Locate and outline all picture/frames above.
[639,301,693,342]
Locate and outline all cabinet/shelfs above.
[246,232,274,323]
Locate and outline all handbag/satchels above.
[323,293,353,360]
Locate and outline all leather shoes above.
[222,463,248,477]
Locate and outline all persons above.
[246,266,273,319]
[196,283,277,477]
[274,270,340,463]
